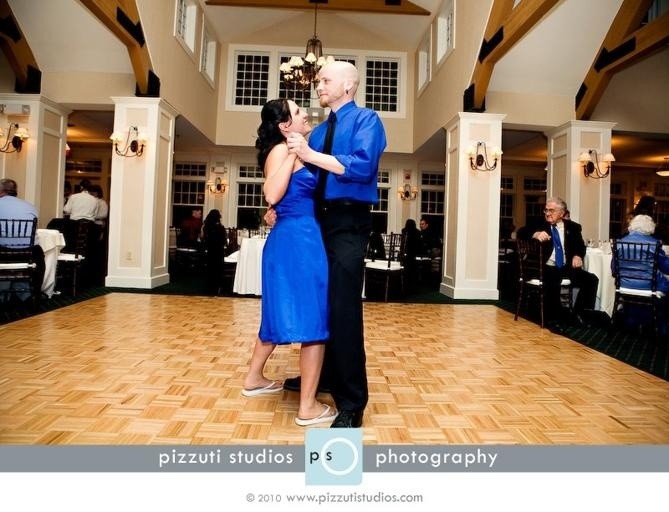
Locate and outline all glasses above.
[543,208,556,213]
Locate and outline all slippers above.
[295,403,338,426]
[241,381,284,397]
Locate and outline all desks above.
[66,123,76,151]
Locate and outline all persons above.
[1,178,47,301]
[610,213,669,334]
[561,210,571,220]
[181,207,203,243]
[363,215,443,297]
[202,209,228,295]
[618,195,656,240]
[64,179,108,241]
[262,60,388,426]
[515,197,599,333]
[242,98,340,427]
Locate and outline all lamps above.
[279,0,336,93]
[204,176,227,193]
[398,183,419,201]
[0,121,31,154]
[655,157,669,176]
[464,140,504,172]
[109,125,147,158]
[579,149,616,179]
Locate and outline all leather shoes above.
[331,414,364,428]
[284,375,301,392]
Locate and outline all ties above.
[312,111,337,222]
[551,224,563,268]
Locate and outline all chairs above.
[171,220,443,303]
[0,214,110,327]
[499,218,669,342]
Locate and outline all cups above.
[584,238,613,254]
[250,226,268,238]
